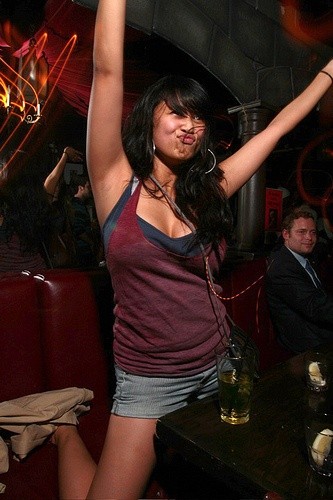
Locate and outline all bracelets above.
[321,71,333,81]
[64,151,69,158]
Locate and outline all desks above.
[156,351,333,500]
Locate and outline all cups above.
[212,344,257,426]
[304,411,333,477]
[304,349,331,393]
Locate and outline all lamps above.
[12,23,51,108]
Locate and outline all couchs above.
[0,260,271,500]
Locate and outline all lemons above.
[311,429,333,466]
[308,362,322,383]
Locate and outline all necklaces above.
[150,175,181,216]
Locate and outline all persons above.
[271,195,333,295]
[0,147,103,276]
[47,0,333,500]
[265,212,333,359]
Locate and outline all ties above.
[305,260,327,296]
